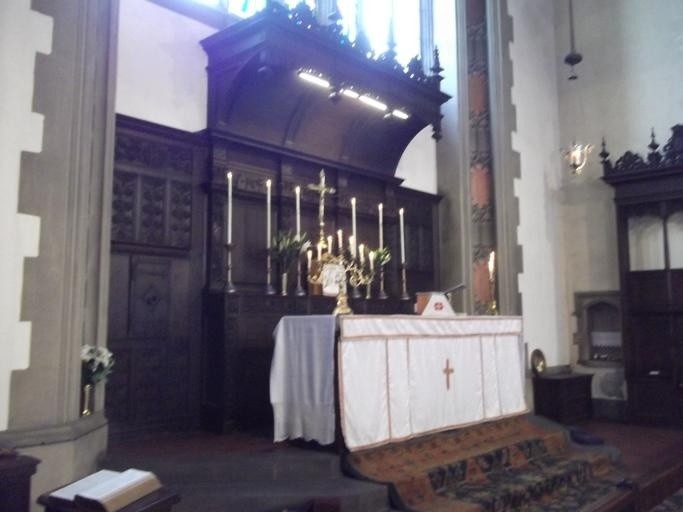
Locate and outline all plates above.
[531,349,548,378]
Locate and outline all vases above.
[281,272,288,297]
[364,281,374,299]
[351,288,363,299]
[82,380,95,421]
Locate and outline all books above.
[48,466,161,511]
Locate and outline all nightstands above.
[536,371,594,426]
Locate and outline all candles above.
[328,235,334,257]
[336,228,348,256]
[226,169,233,243]
[377,203,385,256]
[294,185,302,241]
[398,206,408,266]
[265,179,274,249]
[369,250,376,270]
[348,236,356,256]
[317,242,324,262]
[306,249,313,273]
[358,243,365,261]
[327,235,332,256]
[350,198,358,257]
[360,243,364,265]
[369,250,375,269]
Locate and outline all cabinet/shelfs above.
[600,123,682,427]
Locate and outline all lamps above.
[557,0,596,174]
[295,64,413,124]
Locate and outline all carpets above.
[342,410,642,510]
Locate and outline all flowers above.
[340,252,370,287]
[273,227,311,275]
[348,242,390,283]
[82,341,116,386]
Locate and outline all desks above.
[267,314,532,451]
